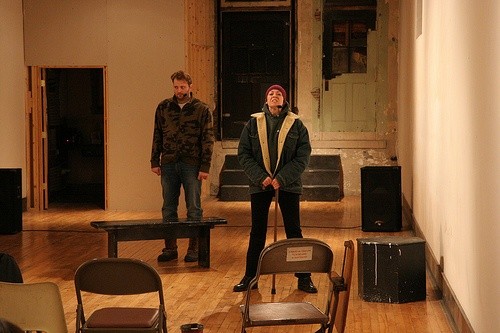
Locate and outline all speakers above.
[0,168,22,234]
[360,166,401,232]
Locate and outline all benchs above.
[90,217,227,270]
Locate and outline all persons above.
[233,84,319,293]
[150,70,215,262]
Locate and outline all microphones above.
[278,106,283,108]
[183,89,189,97]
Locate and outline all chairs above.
[74,257,167,333]
[0,281,68,333]
[240,238,344,333]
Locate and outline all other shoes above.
[158,245,178,261]
[233,278,259,291]
[183,249,198,262]
[297,277,318,293]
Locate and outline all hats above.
[265,84,286,106]
